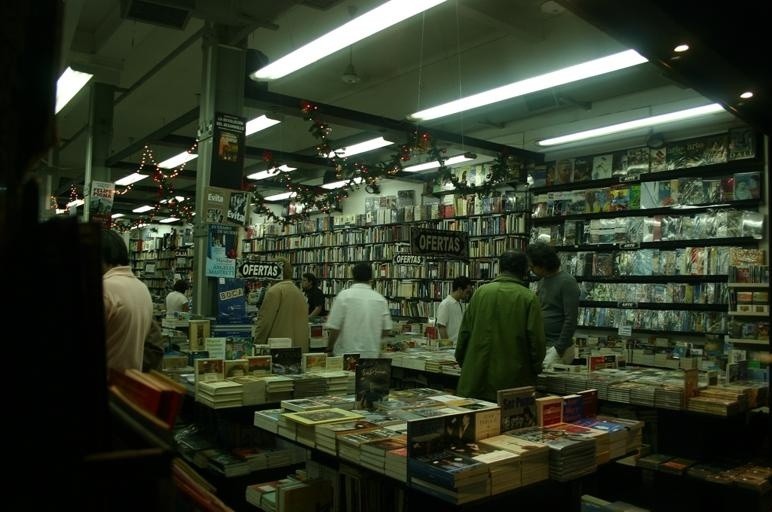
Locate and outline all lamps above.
[158,212,195,224]
[157,149,200,170]
[115,171,149,189]
[111,212,125,220]
[402,152,479,174]
[55,197,85,216]
[55,61,95,119]
[407,42,692,129]
[131,222,150,231]
[245,112,284,138]
[322,135,396,161]
[265,190,300,203]
[247,162,299,182]
[320,174,378,191]
[537,92,755,148]
[251,0,449,88]
[131,193,190,216]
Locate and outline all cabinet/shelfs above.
[165,357,642,510]
[526,156,772,381]
[126,225,197,302]
[240,182,528,331]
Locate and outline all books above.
[450,434,550,496]
[504,422,609,456]
[356,358,391,401]
[246,453,340,512]
[408,449,489,480]
[426,394,499,414]
[325,224,438,246]
[184,281,193,312]
[157,351,209,386]
[728,265,770,284]
[574,335,772,374]
[204,336,255,360]
[576,281,729,304]
[256,371,355,403]
[127,260,153,273]
[324,297,334,312]
[252,344,270,357]
[727,320,770,339]
[153,268,194,281]
[578,389,599,419]
[323,279,355,295]
[526,124,755,189]
[323,242,410,263]
[555,246,766,277]
[322,265,356,278]
[453,259,501,280]
[468,236,526,257]
[531,172,760,217]
[194,356,272,409]
[127,250,157,260]
[134,271,153,279]
[536,395,564,426]
[529,209,765,247]
[608,369,772,416]
[338,462,409,512]
[576,307,728,333]
[728,288,771,313]
[561,394,585,423]
[189,320,210,352]
[153,295,166,315]
[242,232,324,251]
[242,253,263,263]
[497,386,538,432]
[316,190,531,224]
[254,388,366,457]
[264,252,323,265]
[161,311,203,337]
[580,495,649,512]
[411,476,491,504]
[548,457,610,482]
[142,279,152,287]
[438,406,501,446]
[536,353,626,402]
[438,212,529,235]
[129,238,154,252]
[208,436,311,477]
[156,248,194,259]
[174,417,226,469]
[616,444,772,493]
[574,415,645,468]
[393,278,453,301]
[393,300,442,318]
[368,279,393,299]
[268,337,302,374]
[153,258,194,270]
[292,265,323,279]
[333,388,446,483]
[371,263,393,277]
[380,320,462,376]
[242,220,316,235]
[302,353,360,371]
[152,279,167,298]
[393,260,454,279]
[152,227,195,252]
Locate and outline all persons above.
[302,273,326,317]
[436,276,472,341]
[166,281,189,314]
[454,249,546,404]
[251,257,311,367]
[524,241,581,365]
[322,262,393,359]
[82,230,153,385]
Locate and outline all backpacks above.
[143,319,180,374]
